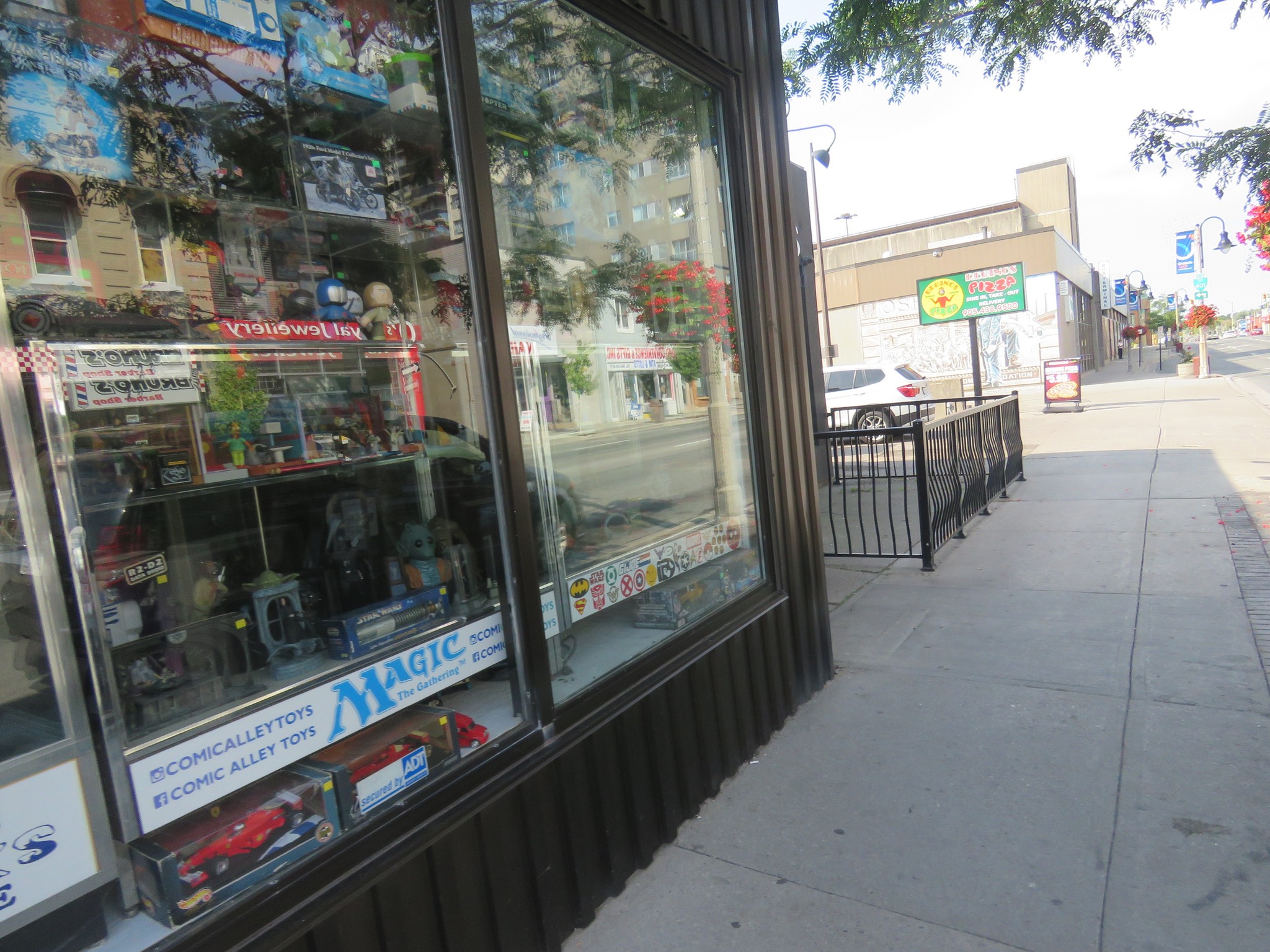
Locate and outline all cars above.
[822,361,936,444]
[0,406,585,689]
[1237,332,1248,337]
[1250,329,1263,335]
[1206,335,1219,340]
[1223,330,1237,339]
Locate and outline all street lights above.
[1195,215,1238,378]
[1174,288,1191,343]
[1126,270,1149,371]
[1137,284,1157,362]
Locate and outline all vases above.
[1178,363,1194,376]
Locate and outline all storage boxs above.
[0,18,135,185]
[323,586,452,659]
[129,762,342,929]
[298,704,463,832]
[283,140,387,220]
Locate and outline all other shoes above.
[1119,357,1123,359]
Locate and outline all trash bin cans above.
[1175,343,1183,352]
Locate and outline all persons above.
[1118,339,1124,359]
[315,154,348,204]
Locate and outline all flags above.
[1129,291,1139,311]
[1176,230,1195,274]
[1114,279,1127,306]
[1167,294,1175,310]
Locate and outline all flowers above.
[1121,326,1139,340]
[631,258,740,375]
[1185,300,1219,329]
[1134,325,1147,336]
[1171,322,1183,333]
[1176,347,1195,364]
